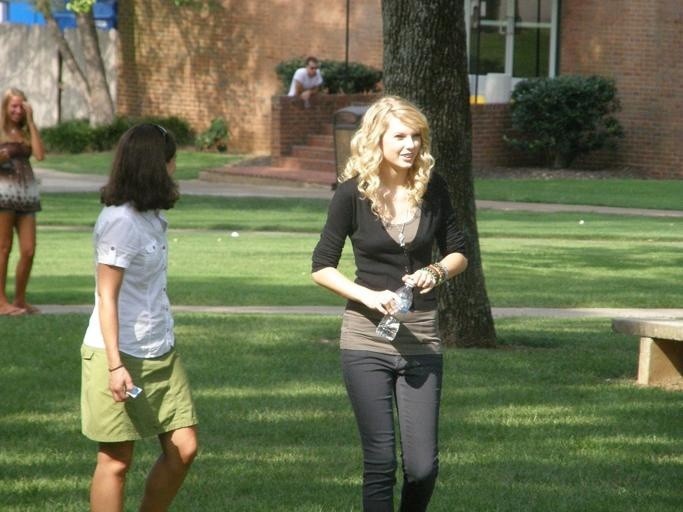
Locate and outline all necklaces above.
[386,194,409,245]
[9,128,17,133]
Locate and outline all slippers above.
[20,306,42,316]
[0,306,27,316]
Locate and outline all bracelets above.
[423,261,448,287]
[108,364,124,373]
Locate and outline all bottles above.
[375,279,415,342]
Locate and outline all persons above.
[0,86,45,316]
[287,57,325,99]
[79,124,198,512]
[312,95,474,512]
